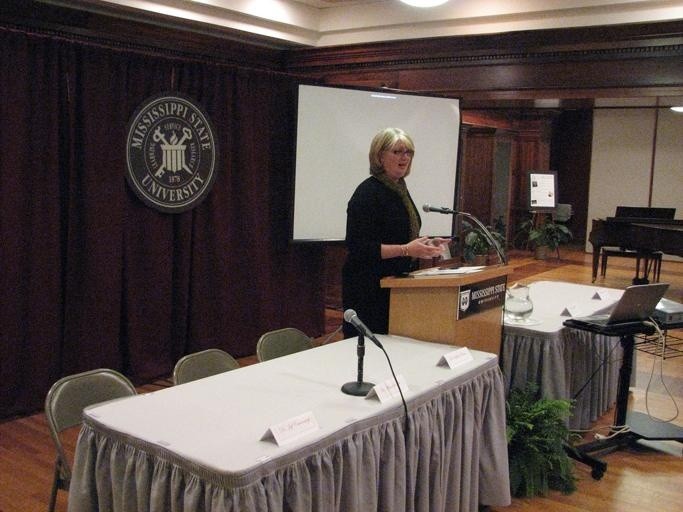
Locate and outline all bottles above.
[504,284,534,322]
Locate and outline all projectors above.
[656,296,683,329]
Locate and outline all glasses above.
[380,149,415,159]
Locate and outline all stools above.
[599,247,663,282]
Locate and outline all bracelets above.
[399,243,408,257]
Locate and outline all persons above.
[341,128,452,339]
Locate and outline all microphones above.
[342,308,385,350]
[422,203,458,215]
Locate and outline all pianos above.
[629,220,683,284]
[590,205,683,278]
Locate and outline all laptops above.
[574,283,669,328]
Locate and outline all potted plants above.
[459,221,506,266]
[523,218,570,261]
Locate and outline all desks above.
[68,329,511,512]
[500,279,640,432]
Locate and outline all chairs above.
[43,367,138,512]
[171,348,240,386]
[254,327,311,363]
